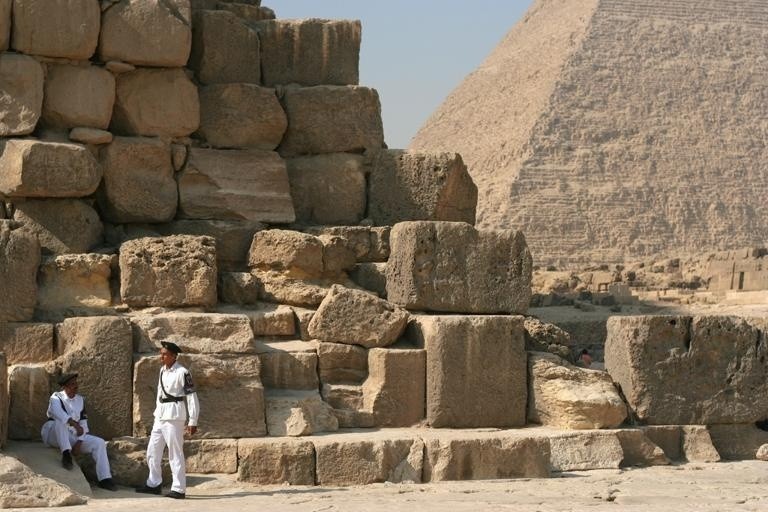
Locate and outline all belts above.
[161,397,184,402]
[47,417,54,420]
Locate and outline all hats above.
[57,373,79,385]
[160,341,183,353]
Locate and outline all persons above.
[41,373,117,491]
[579,349,591,368]
[135,340,200,499]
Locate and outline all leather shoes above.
[136,484,162,495]
[62,451,73,469]
[98,479,117,491]
[165,491,185,499]
